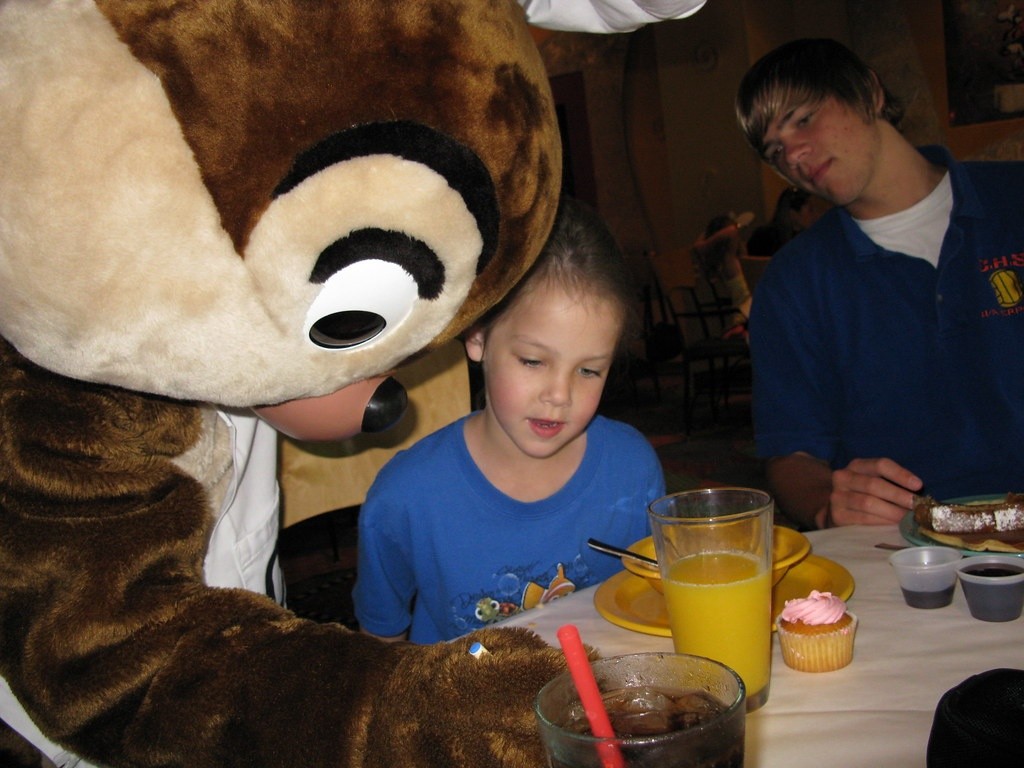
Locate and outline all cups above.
[646,486,774,713]
[534,651,747,768]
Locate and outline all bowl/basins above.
[955,554,1024,623]
[887,546,963,609]
[621,523,811,594]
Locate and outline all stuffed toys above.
[0,2,605,768]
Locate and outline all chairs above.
[279,335,472,628]
[645,244,753,440]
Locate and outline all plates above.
[899,494,1024,559]
[593,552,855,638]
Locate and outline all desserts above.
[777,590,858,672]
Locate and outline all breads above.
[912,493,1024,547]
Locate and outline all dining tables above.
[447,523,1024,768]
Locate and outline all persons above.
[733,37,1024,528]
[351,195,666,648]
[746,189,815,259]
